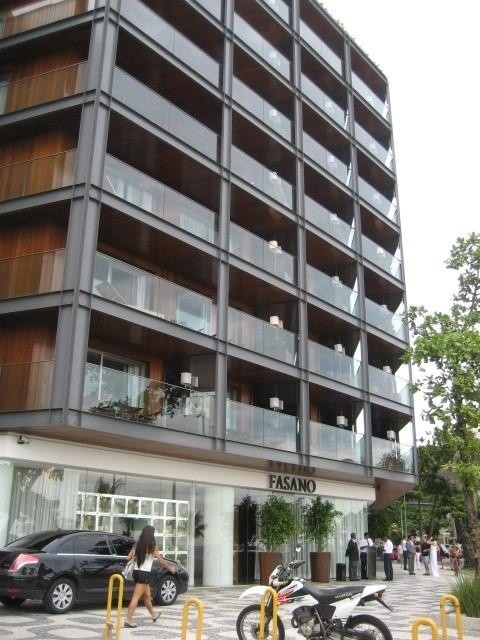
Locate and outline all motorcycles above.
[234,542,396,640]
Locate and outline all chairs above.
[140,383,165,422]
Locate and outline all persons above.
[124,526,175,628]
[345,533,360,581]
[360,532,373,579]
[382,535,393,581]
[397,535,463,577]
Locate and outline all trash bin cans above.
[336,563,346,581]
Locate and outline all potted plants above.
[256,492,299,585]
[303,494,343,583]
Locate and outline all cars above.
[0,529,189,613]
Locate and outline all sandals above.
[153,612,164,622]
[124,622,137,627]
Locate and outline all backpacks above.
[122,558,135,582]
